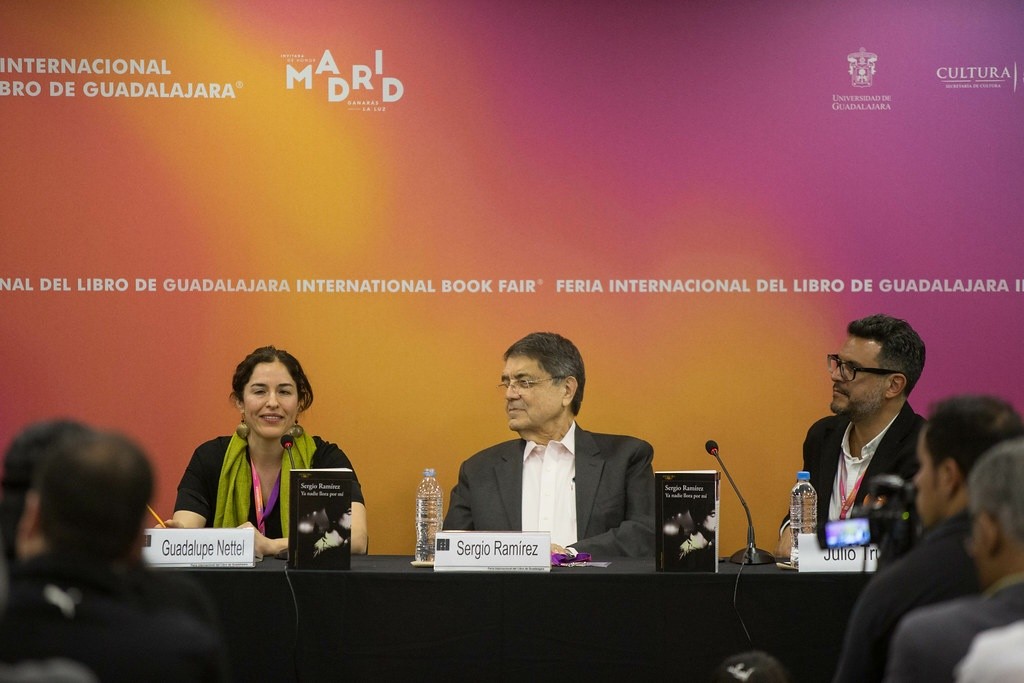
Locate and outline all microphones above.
[280,435,296,469]
[705,440,775,564]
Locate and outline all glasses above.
[496,376,565,392]
[827,355,900,381]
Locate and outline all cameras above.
[817,473,919,551]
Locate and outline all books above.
[652,470,722,573]
[289,468,352,569]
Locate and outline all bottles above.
[415,467,444,563]
[790,470,817,566]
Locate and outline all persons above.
[778,315,928,556]
[720,650,783,683]
[834,394,1024,683]
[0,421,243,683]
[153,345,368,557]
[883,439,1024,683]
[311,494,351,569]
[442,330,654,562]
[674,499,716,572]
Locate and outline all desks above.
[135,552,880,683]
